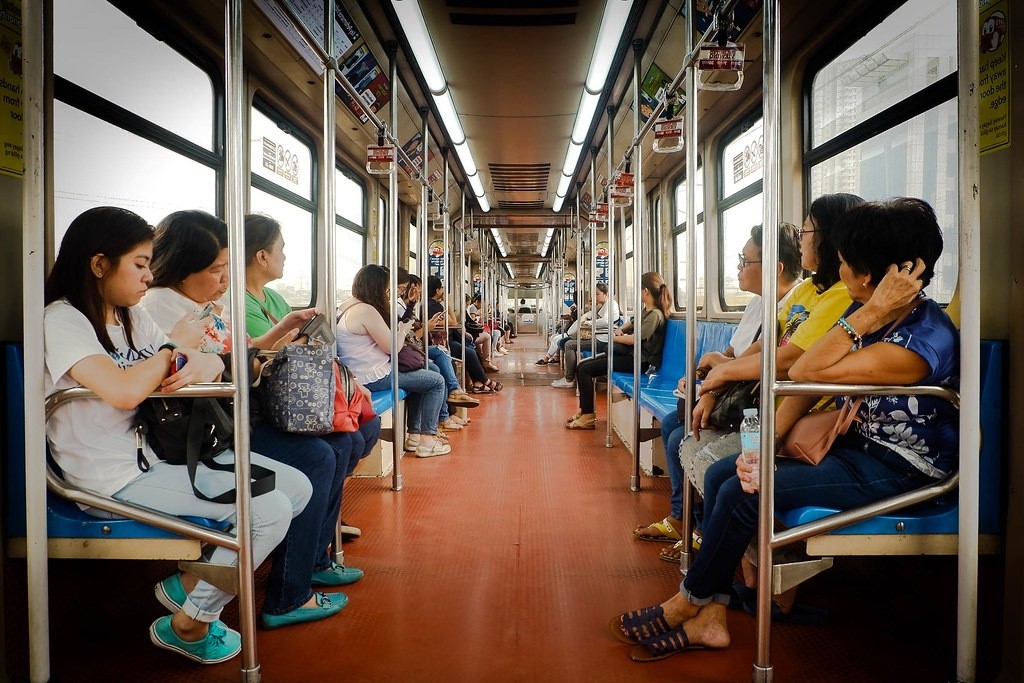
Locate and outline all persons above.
[608,191,960,661]
[551,281,619,387]
[45,206,313,662]
[387,267,480,431]
[140,209,364,627]
[518,298,532,313]
[564,271,672,429]
[336,263,452,457]
[465,332,474,391]
[632,219,807,564]
[678,192,870,617]
[224,214,381,548]
[533,290,590,366]
[464,293,517,372]
[400,273,457,376]
[420,275,503,394]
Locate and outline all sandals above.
[405,329,518,457]
[631,518,683,543]
[659,530,704,563]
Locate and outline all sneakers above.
[149,614,242,664]
[156,573,227,629]
[534,355,596,430]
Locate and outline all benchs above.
[0,342,411,560]
[582,316,1009,595]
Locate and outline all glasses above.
[738,252,761,269]
[798,228,820,241]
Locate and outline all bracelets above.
[399,330,406,337]
[709,389,720,398]
[697,369,707,378]
[837,317,862,349]
[158,342,177,352]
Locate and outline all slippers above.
[629,629,707,661]
[608,602,671,645]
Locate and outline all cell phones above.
[402,318,410,323]
[196,301,214,321]
[290,313,318,342]
[434,310,447,322]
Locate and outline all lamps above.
[391,0,515,280]
[534,0,634,280]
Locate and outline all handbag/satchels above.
[399,339,426,373]
[556,315,573,334]
[677,368,755,433]
[775,393,863,467]
[427,331,447,348]
[604,334,647,358]
[249,338,336,433]
[330,356,377,431]
[579,321,592,340]
[133,391,276,505]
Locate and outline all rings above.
[902,265,911,271]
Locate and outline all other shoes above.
[730,577,804,626]
[310,559,364,587]
[339,519,361,542]
[261,590,349,632]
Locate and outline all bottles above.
[740,408,760,489]
[696,379,701,400]
